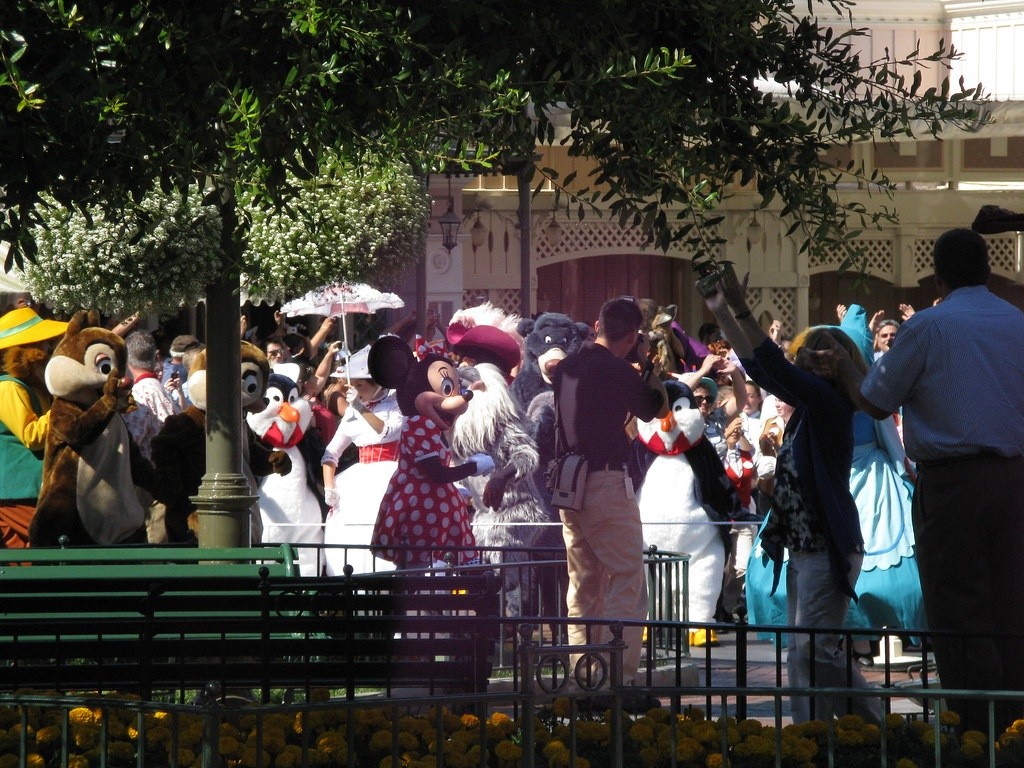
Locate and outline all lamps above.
[440,177,460,253]
[471,211,486,249]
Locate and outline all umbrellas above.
[279,279,405,387]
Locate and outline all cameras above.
[338,342,345,350]
[286,327,297,333]
[624,332,644,364]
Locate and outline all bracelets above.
[736,310,753,320]
[740,430,744,437]
[777,343,785,349]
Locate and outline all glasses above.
[266,349,284,357]
[694,396,714,404]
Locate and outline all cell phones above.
[170,373,178,387]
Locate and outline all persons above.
[696,264,889,730]
[803,226,1023,733]
[555,299,670,714]
[503,312,593,638]
[0,294,973,645]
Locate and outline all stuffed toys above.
[151,337,292,704]
[446,300,547,637]
[29,309,160,705]
[744,304,931,665]
[367,335,492,697]
[0,306,73,566]
[633,380,740,646]
[245,374,323,581]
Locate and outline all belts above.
[588,461,625,473]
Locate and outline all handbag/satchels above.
[551,455,589,512]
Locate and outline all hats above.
[329,344,372,378]
[172,334,200,352]
[700,376,717,403]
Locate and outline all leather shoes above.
[855,638,874,667]
[901,646,923,656]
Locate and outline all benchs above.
[0,545,329,662]
